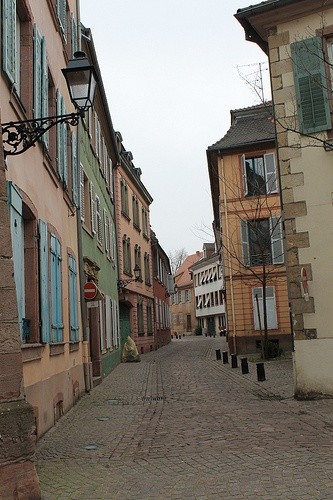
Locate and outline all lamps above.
[116,264,141,289]
[0,50,99,156]
[165,284,178,297]
